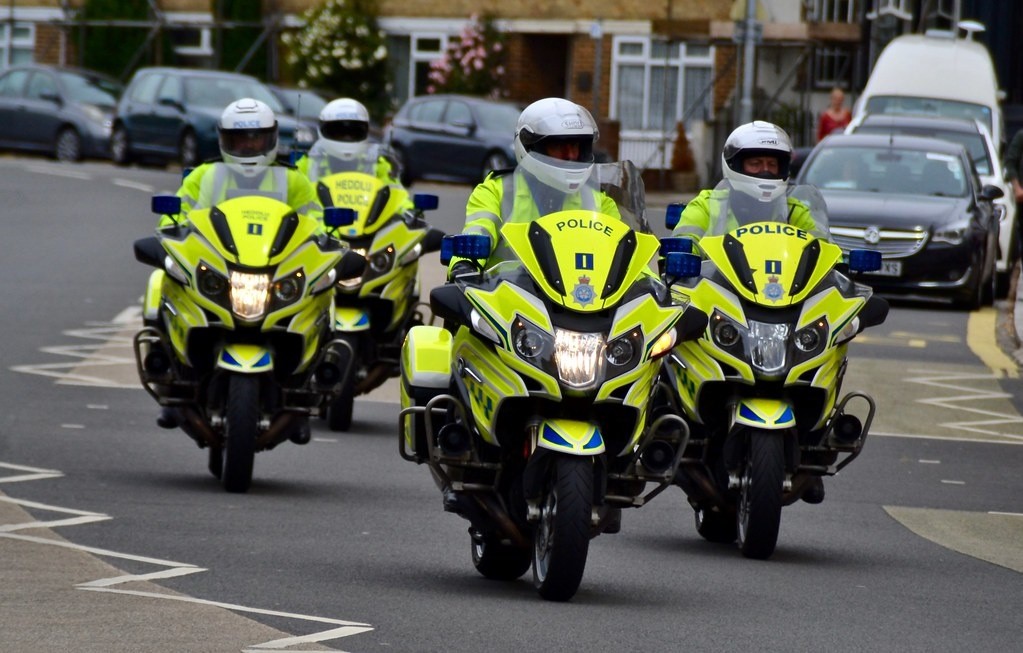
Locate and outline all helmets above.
[317,98,369,161]
[515,97,600,194]
[217,97,279,178]
[721,120,795,202]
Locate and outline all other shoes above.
[601,508,621,533]
[801,477,825,503]
[156,407,179,428]
[443,488,466,512]
[290,418,311,444]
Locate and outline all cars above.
[0,63,122,163]
[386,95,522,193]
[844,111,1018,300]
[795,134,1004,313]
[109,67,318,167]
[268,86,410,184]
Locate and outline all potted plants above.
[672,123,699,193]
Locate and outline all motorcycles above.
[397,152,709,599]
[636,182,888,559]
[133,163,350,491]
[309,144,447,430]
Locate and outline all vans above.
[851,34,1006,157]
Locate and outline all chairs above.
[878,165,920,194]
[917,161,961,195]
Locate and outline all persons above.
[817,87,851,143]
[999,129,1023,261]
[647,117,829,506]
[142,97,330,410]
[435,97,671,284]
[294,97,426,376]
